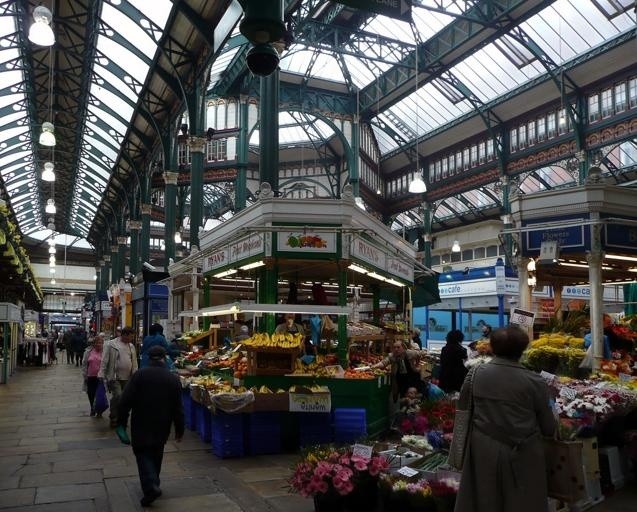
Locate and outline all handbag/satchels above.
[448,363,480,470]
[95,377,109,416]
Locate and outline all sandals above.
[111,418,117,428]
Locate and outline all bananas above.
[242,332,304,348]
[289,384,330,393]
[293,355,338,378]
[190,376,218,390]
[212,384,285,394]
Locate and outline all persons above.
[448,323,555,512]
[602,314,613,329]
[468,340,479,351]
[0,319,175,429]
[274,313,307,354]
[391,387,420,431]
[119,344,186,506]
[213,313,252,345]
[371,339,442,409]
[412,326,423,350]
[440,329,470,392]
[481,325,496,339]
[419,317,446,332]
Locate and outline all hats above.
[148,345,166,360]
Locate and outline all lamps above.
[408,36,427,194]
[452,198,461,253]
[28,0,58,285]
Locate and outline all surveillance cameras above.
[245,44,280,75]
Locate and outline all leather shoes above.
[141,486,161,506]
[91,410,95,416]
